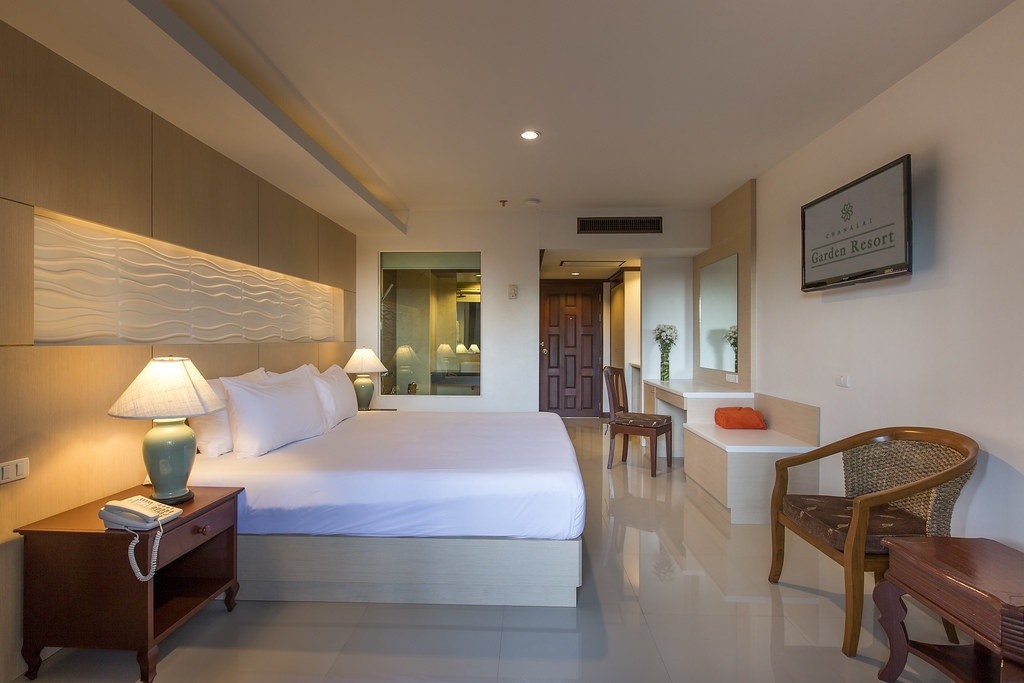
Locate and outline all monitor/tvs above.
[799,154,912,293]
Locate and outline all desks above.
[682,423,821,525]
[871,536,1024,683]
[643,378,754,459]
[629,361,640,410]
[11,485,246,683]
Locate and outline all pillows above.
[188,361,360,460]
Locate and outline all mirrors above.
[379,250,482,397]
[699,253,738,373]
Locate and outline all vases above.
[659,351,670,383]
[734,353,738,373]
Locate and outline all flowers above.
[723,325,738,353]
[653,324,678,354]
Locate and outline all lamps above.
[343,346,389,410]
[107,357,229,505]
[388,343,479,375]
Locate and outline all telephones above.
[98,494,183,532]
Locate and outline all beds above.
[145,413,586,607]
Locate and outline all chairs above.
[768,426,979,658]
[603,366,673,477]
